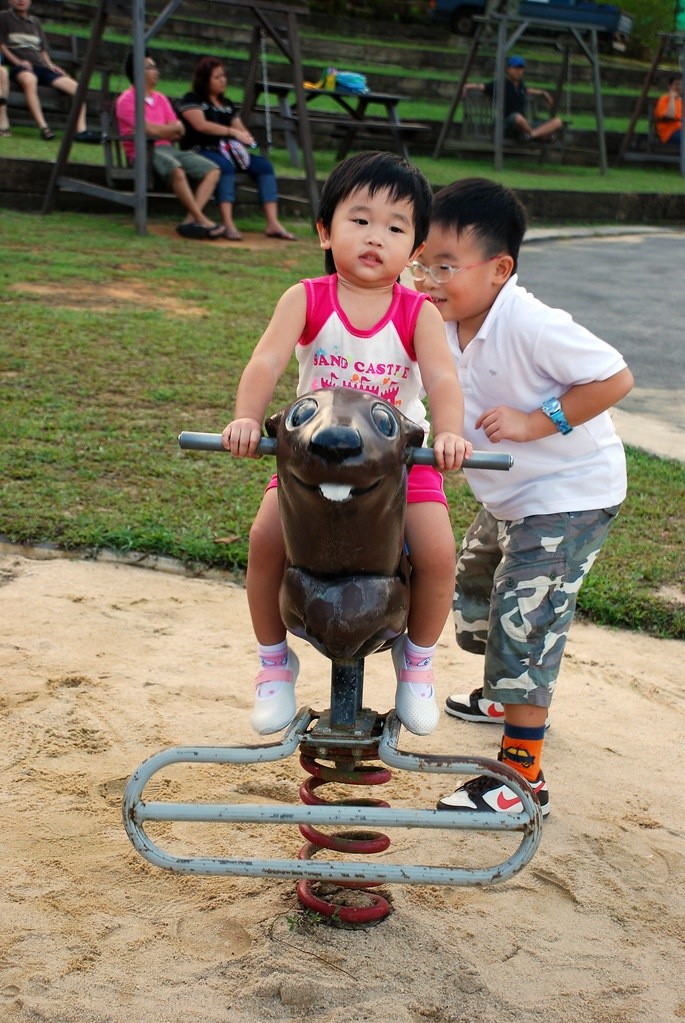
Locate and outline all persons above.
[115,55,226,239]
[653,79,683,142]
[221,152,456,737]
[0,0,102,144]
[464,56,562,142]
[408,178,633,818]
[177,59,297,242]
[0,65,13,138]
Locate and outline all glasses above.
[409,255,503,283]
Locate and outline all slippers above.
[267,230,297,240]
[177,223,209,239]
[195,224,226,237]
[224,231,242,241]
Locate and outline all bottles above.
[325,65,337,91]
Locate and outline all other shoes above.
[336,71,368,95]
[0,129,12,138]
[392,633,440,736]
[41,128,54,138]
[252,646,299,736]
[519,133,531,142]
[540,135,559,144]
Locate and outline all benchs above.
[8,32,77,113]
[242,103,432,134]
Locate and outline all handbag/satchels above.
[219,138,250,170]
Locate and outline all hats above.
[505,56,525,66]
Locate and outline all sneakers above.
[444,686,551,729]
[437,774,549,818]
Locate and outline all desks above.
[250,80,413,172]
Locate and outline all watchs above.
[542,397,572,436]
